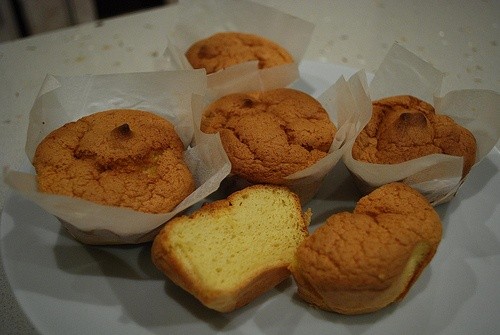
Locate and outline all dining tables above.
[0,0,500,335]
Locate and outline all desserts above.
[32,109,194,245]
[288,182,443,315]
[150,183,312,313]
[200,87,338,208]
[184,32,293,87]
[352,94,477,207]
